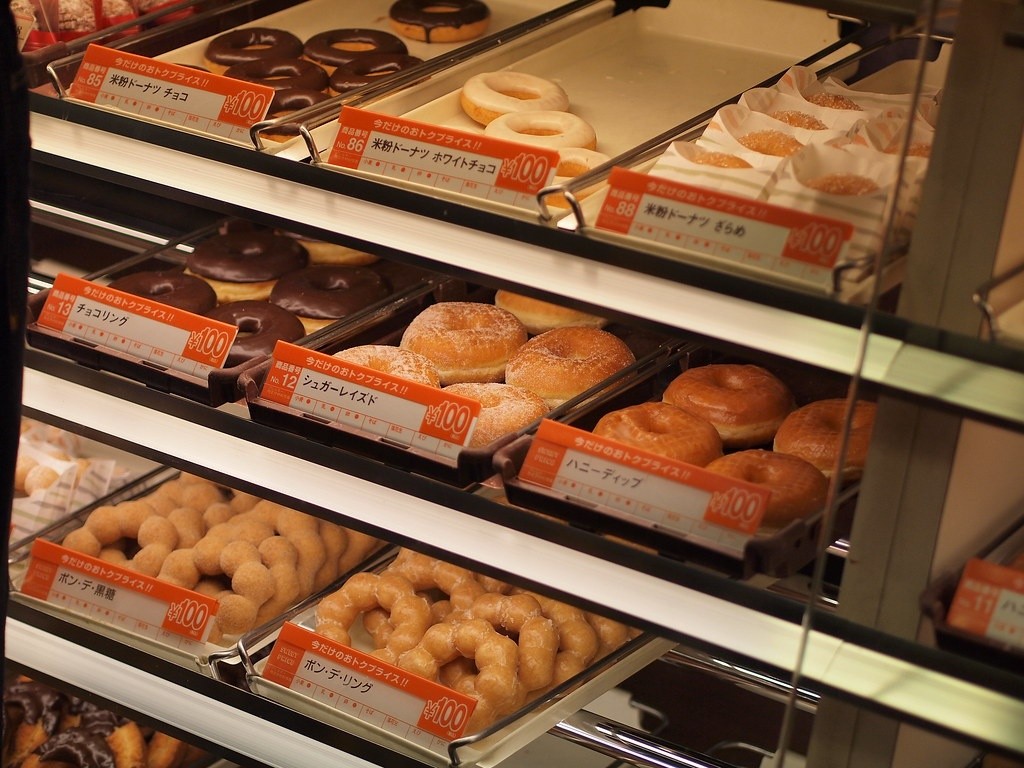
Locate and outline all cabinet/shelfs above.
[0,0,1024,768]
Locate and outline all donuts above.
[1,0,946,768]
